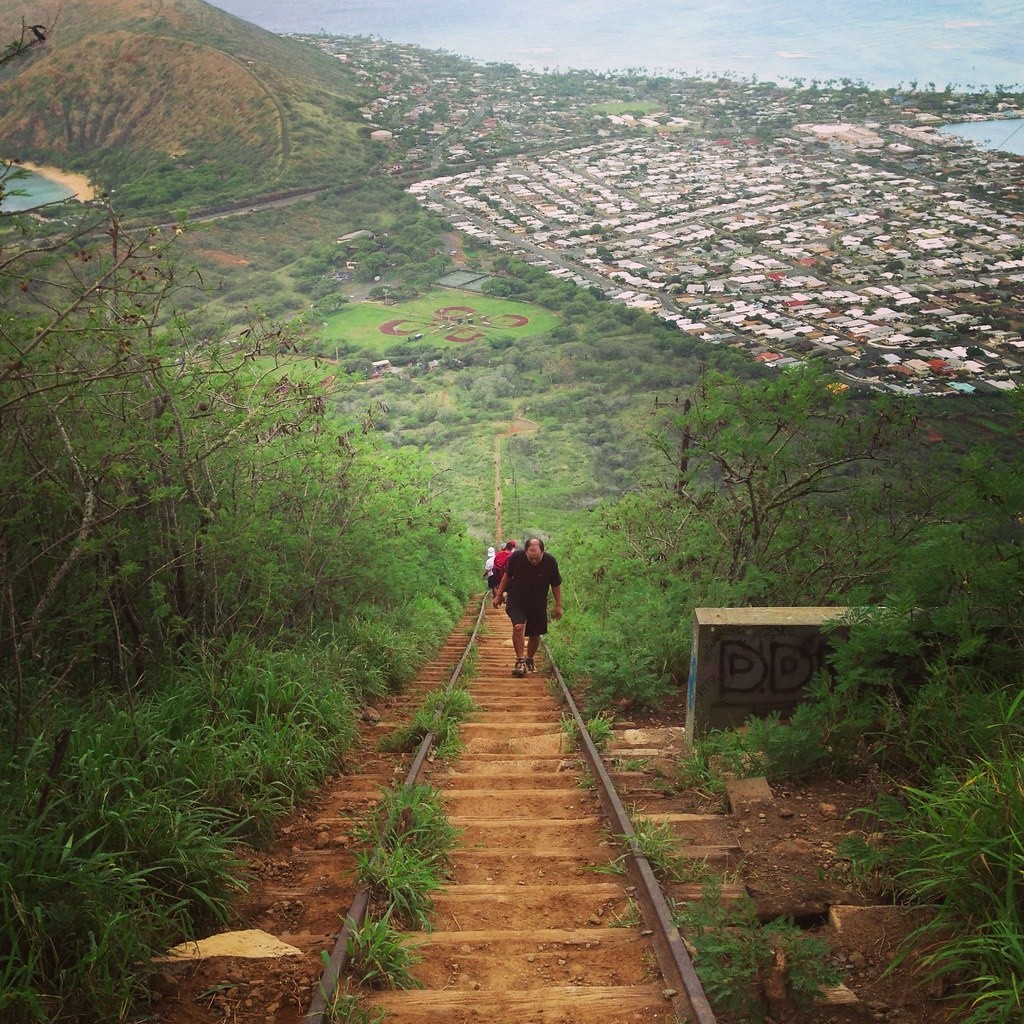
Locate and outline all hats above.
[508,540,516,548]
[487,546,495,557]
[501,543,507,551]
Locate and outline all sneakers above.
[512,659,527,678]
[525,657,539,675]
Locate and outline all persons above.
[492,537,562,677]
[483,541,517,605]
